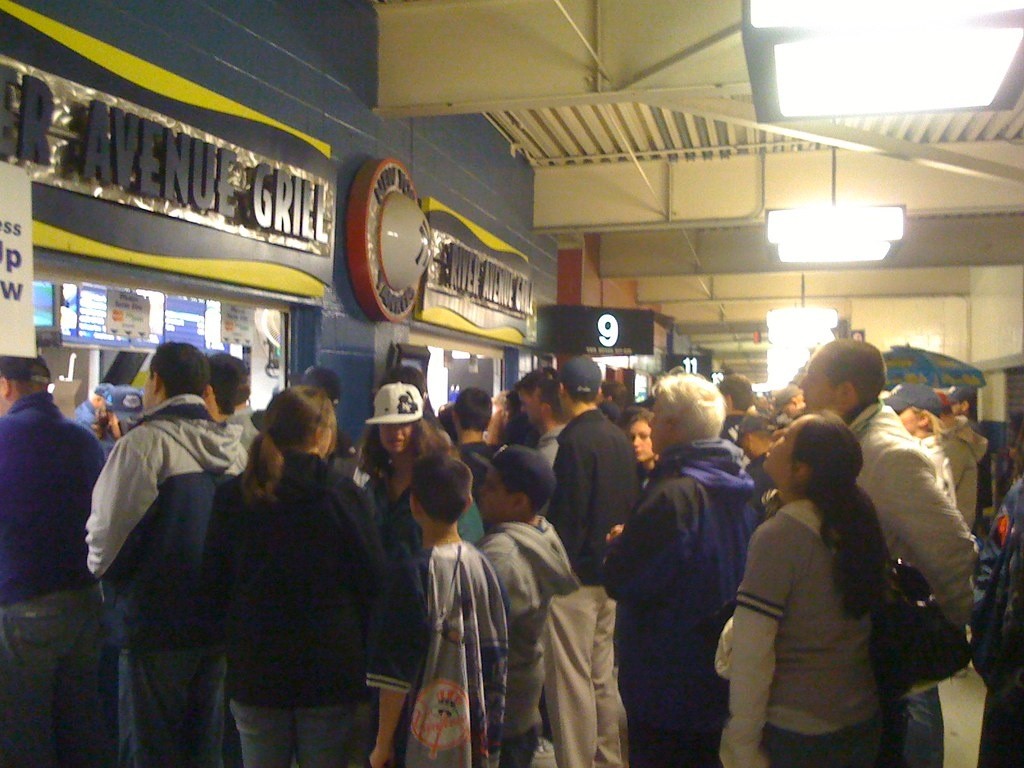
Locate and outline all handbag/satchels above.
[867,560,972,702]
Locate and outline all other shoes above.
[532,737,555,759]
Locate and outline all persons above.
[0,337,1024,768]
[720,412,893,768]
[600,370,759,768]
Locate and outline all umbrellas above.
[881,344,987,392]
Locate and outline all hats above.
[946,385,974,403]
[776,384,800,406]
[364,381,424,425]
[881,383,945,417]
[0,354,51,383]
[303,365,346,397]
[95,382,115,399]
[468,446,556,507]
[106,384,144,414]
[557,355,602,393]
